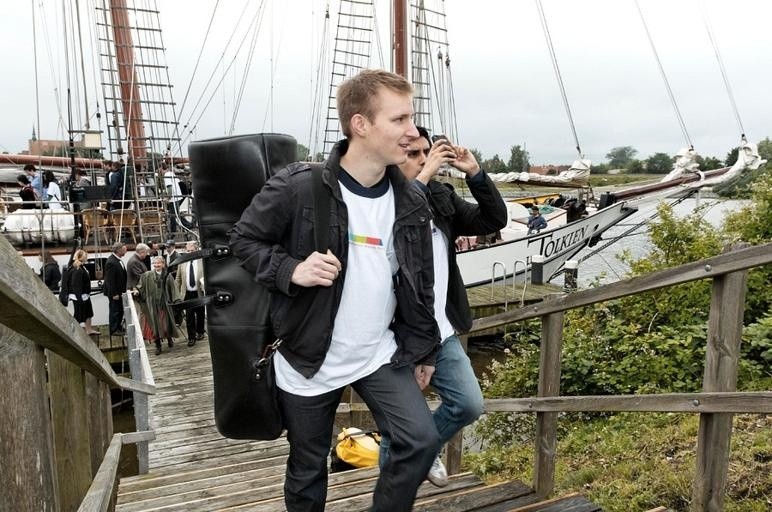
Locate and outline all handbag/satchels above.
[331,427,381,474]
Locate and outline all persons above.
[15,158,195,214]
[396,124,509,489]
[28,239,208,355]
[527,206,548,234]
[474,229,503,248]
[226,67,442,512]
[552,193,587,223]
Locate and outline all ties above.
[190,259,195,287]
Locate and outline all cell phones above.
[432,135,458,157]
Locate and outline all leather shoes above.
[155,333,204,356]
[112,324,126,336]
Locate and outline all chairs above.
[81,206,165,245]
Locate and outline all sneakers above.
[427,456,449,487]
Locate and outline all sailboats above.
[0,0,768,327]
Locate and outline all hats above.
[165,240,175,247]
[112,242,125,252]
